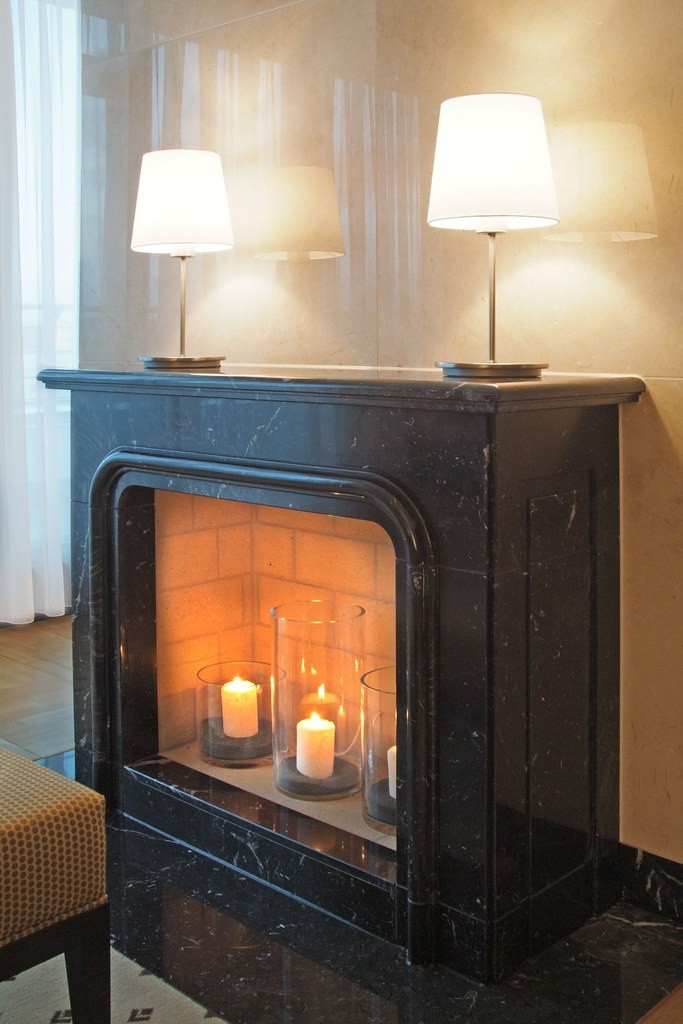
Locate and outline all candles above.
[297,719,336,779]
[387,745,397,798]
[221,680,258,737]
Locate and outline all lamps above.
[425,93,560,378]
[131,149,234,370]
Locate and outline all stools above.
[0,743,111,1024]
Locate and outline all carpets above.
[0,945,231,1024]
